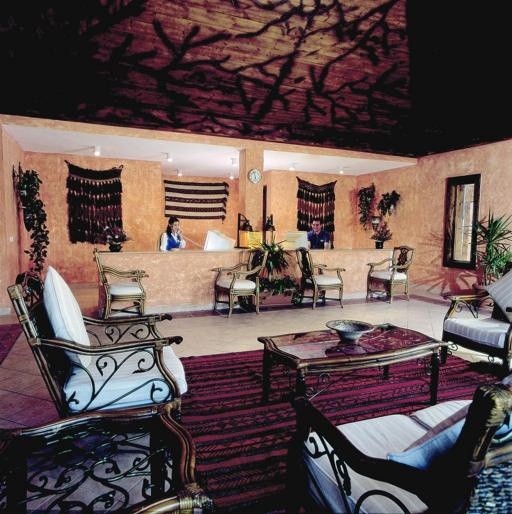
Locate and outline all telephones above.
[176,225,183,234]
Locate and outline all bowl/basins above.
[325,319,376,341]
[324,341,368,357]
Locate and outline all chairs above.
[440,261,511,380]
[8,269,183,422]
[211,249,268,318]
[92,247,149,320]
[365,245,415,305]
[282,383,512,513]
[295,247,346,310]
[1,400,216,514]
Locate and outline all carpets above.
[177,348,499,514]
[0,323,23,364]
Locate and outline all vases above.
[375,240,383,249]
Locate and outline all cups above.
[324,241,332,250]
[308,240,312,250]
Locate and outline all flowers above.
[102,228,127,245]
[369,215,393,241]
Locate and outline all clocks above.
[248,169,262,183]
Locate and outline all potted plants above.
[358,183,375,230]
[377,190,400,216]
[370,216,383,231]
[469,207,512,308]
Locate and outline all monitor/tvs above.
[203,229,235,251]
[282,231,308,250]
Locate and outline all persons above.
[307,217,334,250]
[159,216,187,252]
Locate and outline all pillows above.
[42,264,92,368]
[386,374,510,470]
[481,269,510,322]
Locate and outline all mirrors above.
[442,174,482,271]
